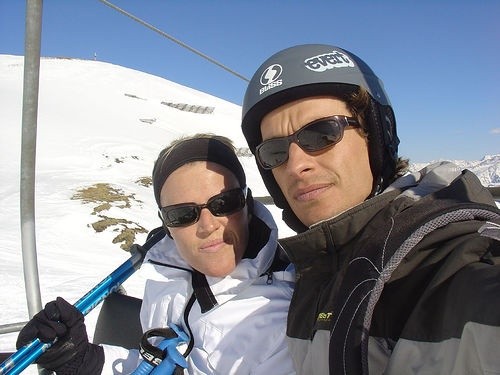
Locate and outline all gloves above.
[15,296,105,375]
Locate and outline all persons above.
[16,133,296,375]
[242,44,500,375]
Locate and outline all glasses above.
[158,184,248,228]
[254,115,362,172]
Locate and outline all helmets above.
[241,43,400,210]
